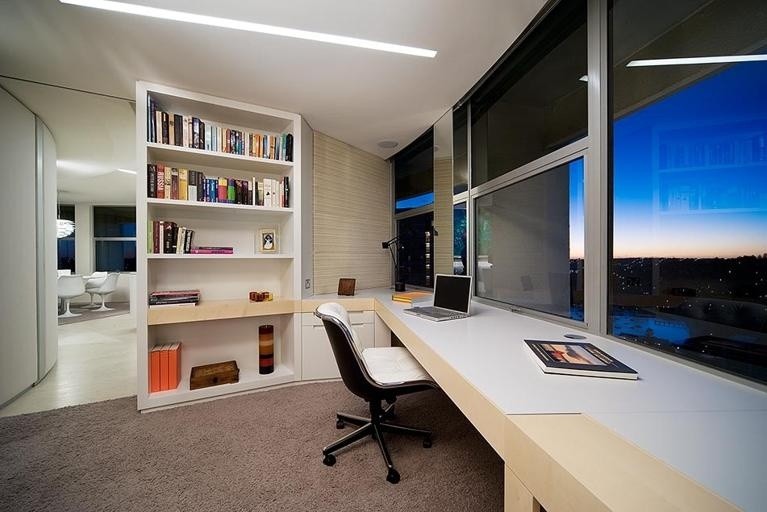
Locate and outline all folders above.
[169,340,182,389]
[160,342,174,391]
[149,343,164,393]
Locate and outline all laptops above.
[403,274,473,322]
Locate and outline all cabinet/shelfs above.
[301,313,392,383]
[134,81,301,415]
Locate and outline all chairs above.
[313,303,441,483]
[86,272,120,311]
[56,275,86,317]
[57,269,71,278]
[80,271,109,309]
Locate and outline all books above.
[148,219,234,254]
[149,288,201,309]
[391,291,433,304]
[525,339,640,380]
[148,163,290,207]
[150,342,182,392]
[148,93,295,162]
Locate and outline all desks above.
[375,289,766,510]
[78,274,104,285]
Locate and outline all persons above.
[263,235,273,248]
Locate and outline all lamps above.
[56,219,74,238]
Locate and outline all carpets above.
[0,380,504,511]
